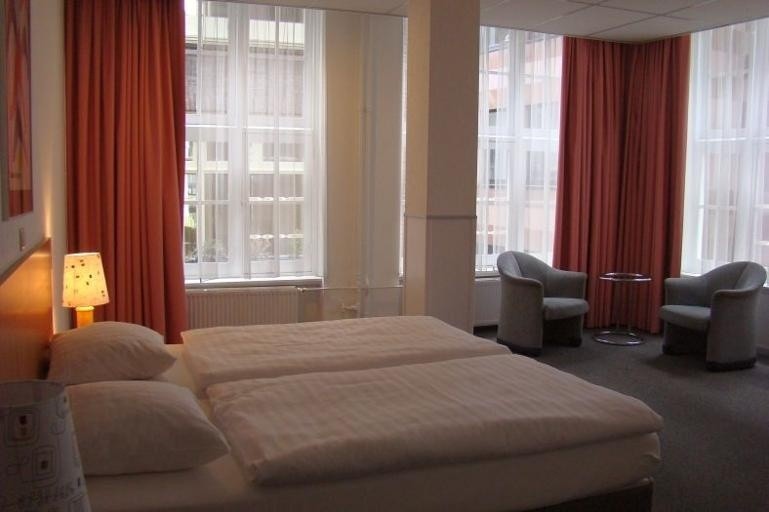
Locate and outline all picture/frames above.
[0,0,34,223]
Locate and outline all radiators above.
[184,286,299,330]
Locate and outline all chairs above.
[664,262,767,369]
[494,249,590,352]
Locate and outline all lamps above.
[60,250,110,332]
[1,378,89,512]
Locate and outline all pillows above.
[65,382,232,480]
[46,321,178,385]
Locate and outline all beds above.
[0,236,663,510]
